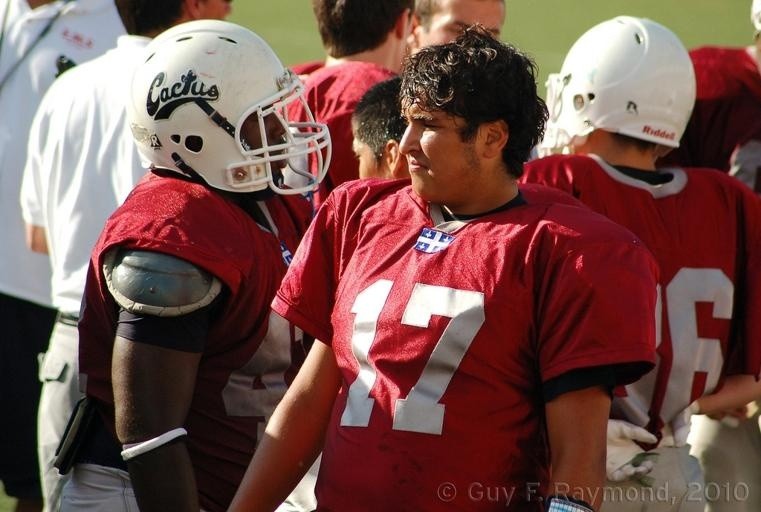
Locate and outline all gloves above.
[668,404,702,449]
[604,415,660,487]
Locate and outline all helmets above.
[122,14,299,199]
[554,13,702,152]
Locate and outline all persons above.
[2,0,761,510]
